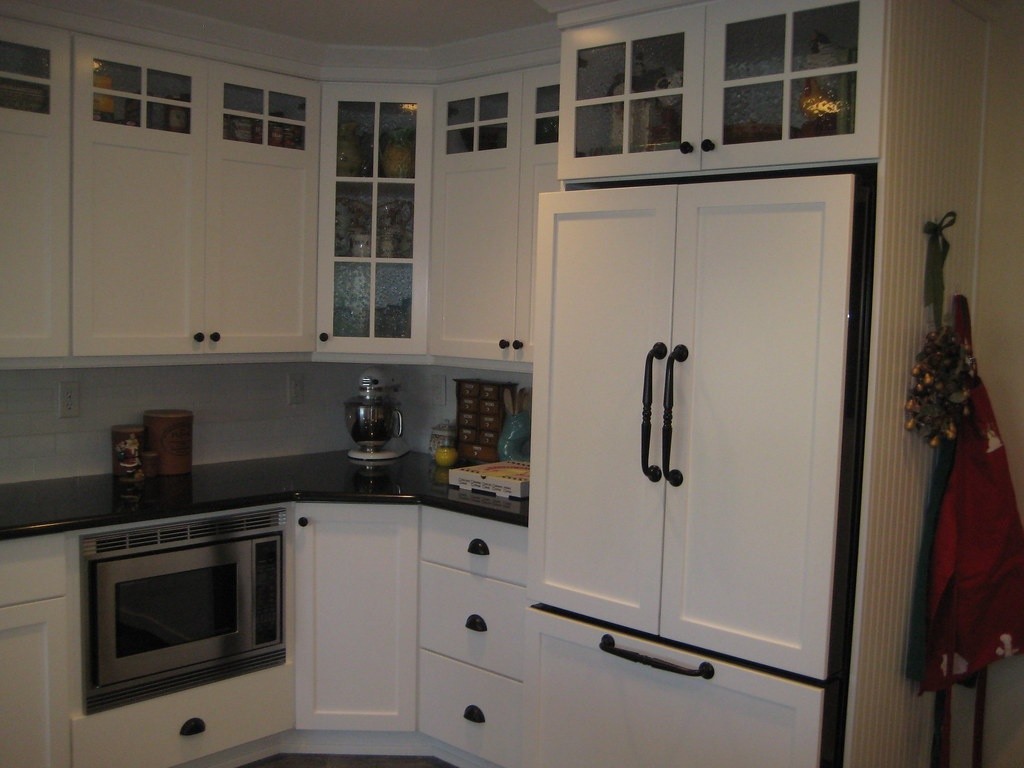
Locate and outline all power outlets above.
[57,382,79,418]
[432,375,446,407]
[286,373,305,406]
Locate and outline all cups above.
[348,233,369,257]
[430,419,456,460]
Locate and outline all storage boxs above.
[449,461,530,498]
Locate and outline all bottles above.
[378,127,413,178]
[338,121,362,176]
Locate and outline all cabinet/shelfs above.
[526,0,993,768]
[417,501,529,768]
[0,0,73,370]
[281,500,421,756]
[0,532,74,768]
[73,663,295,768]
[72,1,322,368]
[428,46,561,373]
[452,378,519,463]
[312,80,434,365]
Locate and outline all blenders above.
[343,376,410,460]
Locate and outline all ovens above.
[80,507,287,716]
